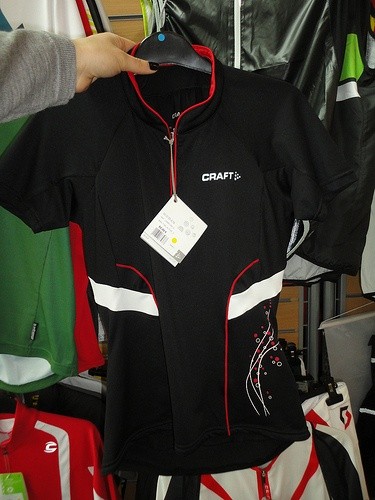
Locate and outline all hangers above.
[0,388,16,414]
[133,31,212,74]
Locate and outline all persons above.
[0,26,163,125]
